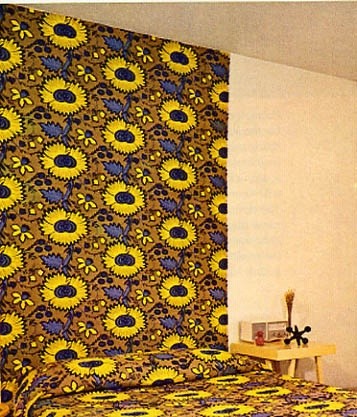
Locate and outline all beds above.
[7,369,357,416]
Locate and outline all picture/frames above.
[241,320,267,343]
[265,319,290,342]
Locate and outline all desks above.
[230,339,336,385]
